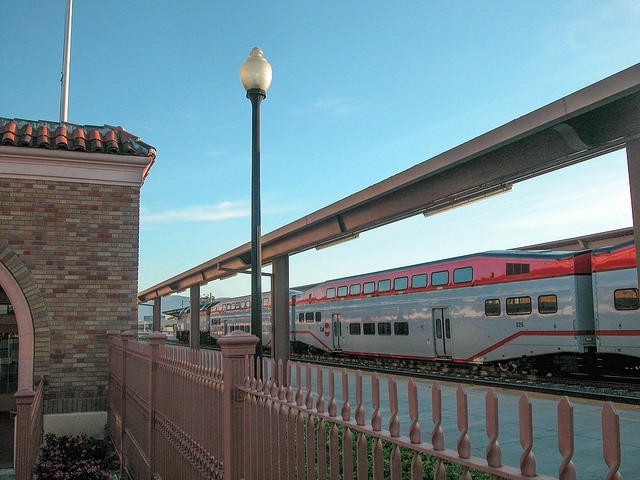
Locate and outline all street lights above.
[239,46,274,382]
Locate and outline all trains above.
[170,240,637,376]
